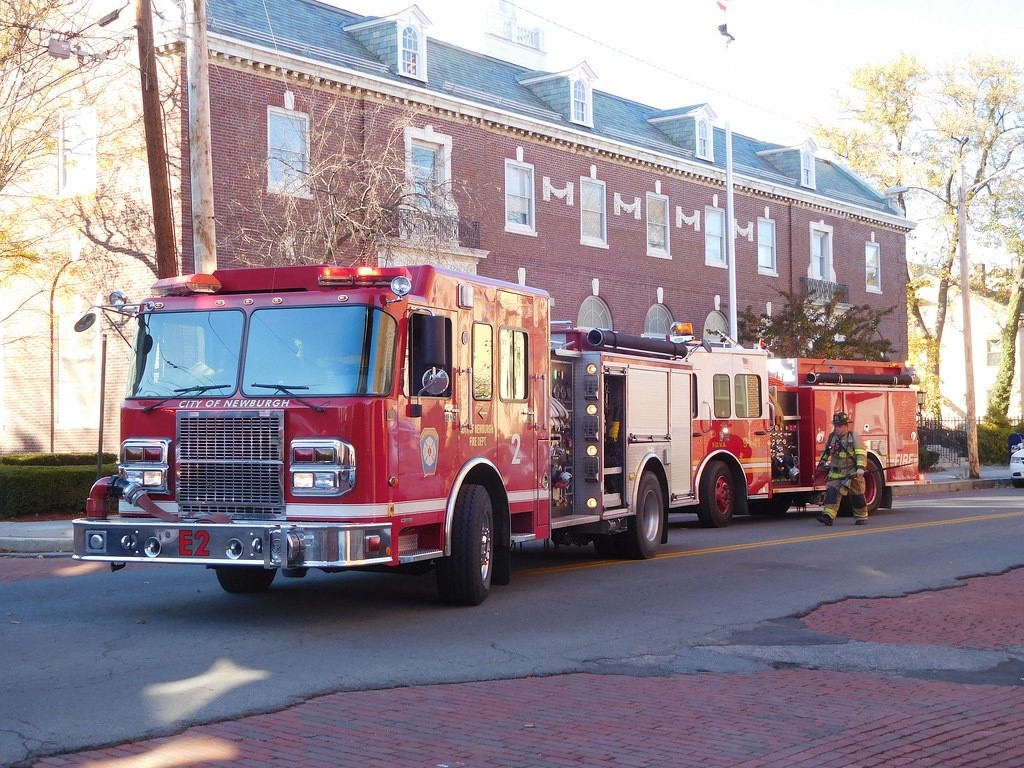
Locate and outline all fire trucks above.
[666,308,930,527]
[68,254,695,610]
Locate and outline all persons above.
[817,412,869,526]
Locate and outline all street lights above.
[73,289,135,477]
[49,259,85,452]
[883,182,980,479]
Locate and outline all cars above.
[1009,443,1024,487]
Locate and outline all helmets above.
[832,411,851,424]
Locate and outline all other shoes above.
[855,519,868,525]
[817,514,833,526]
